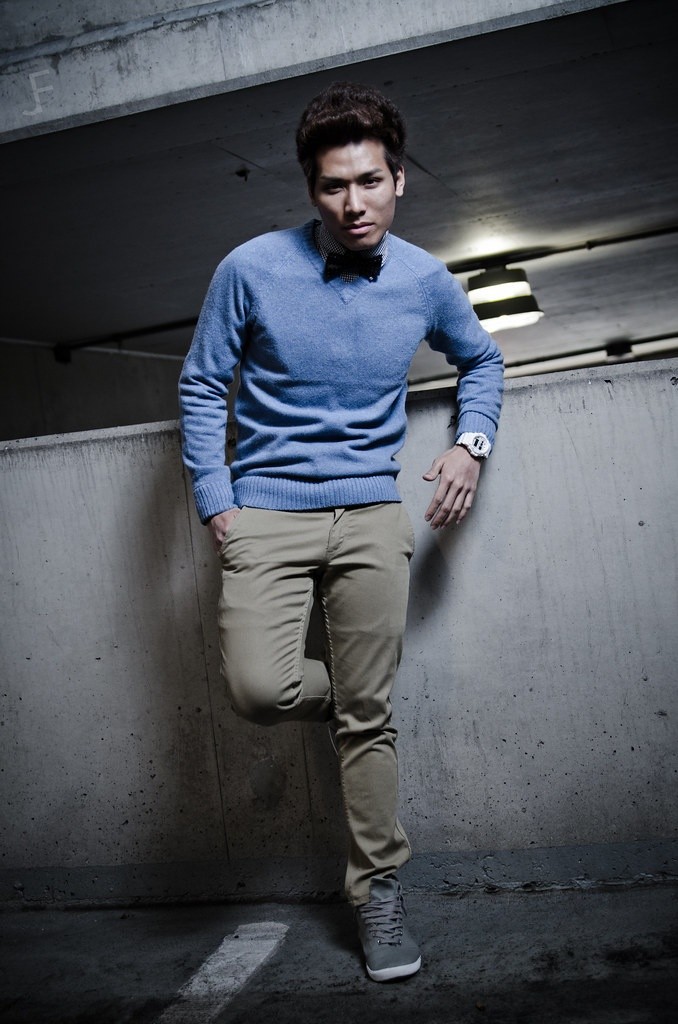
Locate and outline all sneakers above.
[355,877,420,981]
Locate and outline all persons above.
[176,78,504,982]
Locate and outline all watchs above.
[455,432,491,460]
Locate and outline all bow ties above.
[320,253,382,283]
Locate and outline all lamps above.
[467,263,544,334]
